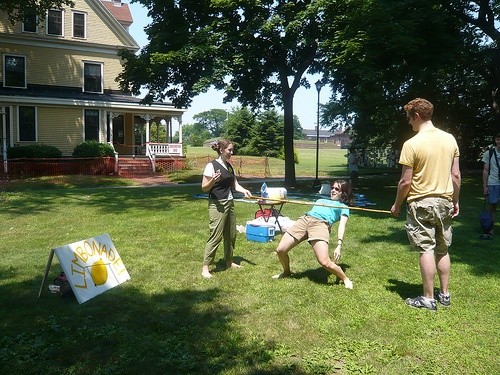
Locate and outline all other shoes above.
[344,278,354,290]
[435,290,451,307]
[270,271,292,279]
[201,267,212,278]
[480,229,494,239]
[227,261,242,268]
[404,295,437,312]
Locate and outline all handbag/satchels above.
[480,210,493,233]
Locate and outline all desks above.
[256,201,286,236]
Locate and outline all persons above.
[479,132,500,240]
[348,147,360,190]
[272,180,353,290]
[390,97,461,312]
[202,139,251,278]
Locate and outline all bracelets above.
[337,239,343,247]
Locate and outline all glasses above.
[331,187,341,191]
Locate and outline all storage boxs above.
[245,223,275,242]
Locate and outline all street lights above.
[315,79,324,180]
